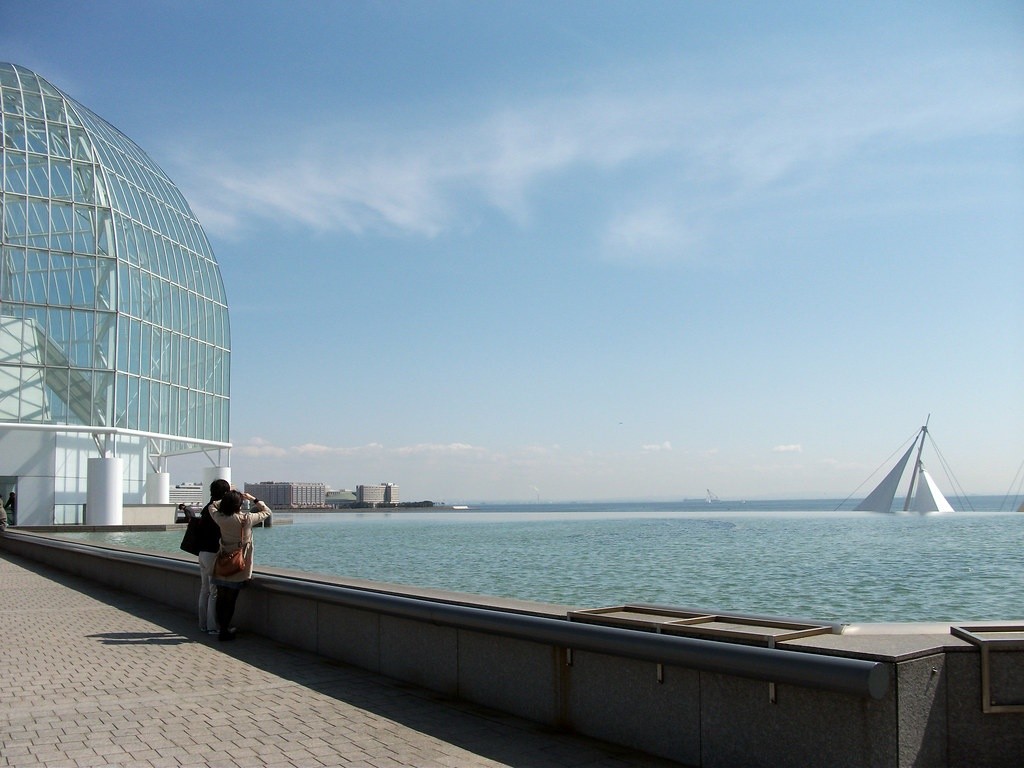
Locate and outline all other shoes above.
[217,631,236,642]
[208,629,220,635]
[200,626,208,631]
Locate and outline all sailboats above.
[705,489,722,504]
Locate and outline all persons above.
[199,479,237,635]
[208,490,272,641]
[4,491,16,525]
[179,504,195,523]
[0,495,7,532]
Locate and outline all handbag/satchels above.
[214,546,245,578]
[180,515,202,556]
